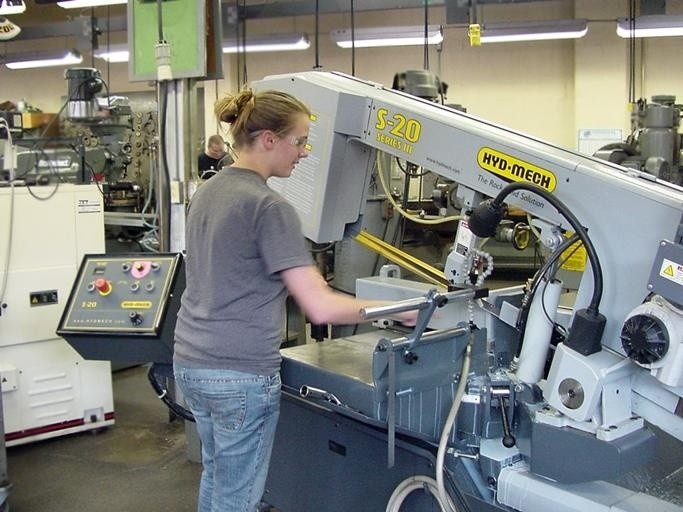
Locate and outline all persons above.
[196,132,233,182]
[169,85,441,512]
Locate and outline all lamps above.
[0,0,129,72]
[222,13,312,55]
[470,12,589,46]
[329,7,444,50]
[616,10,681,43]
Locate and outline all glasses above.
[265,128,310,150]
